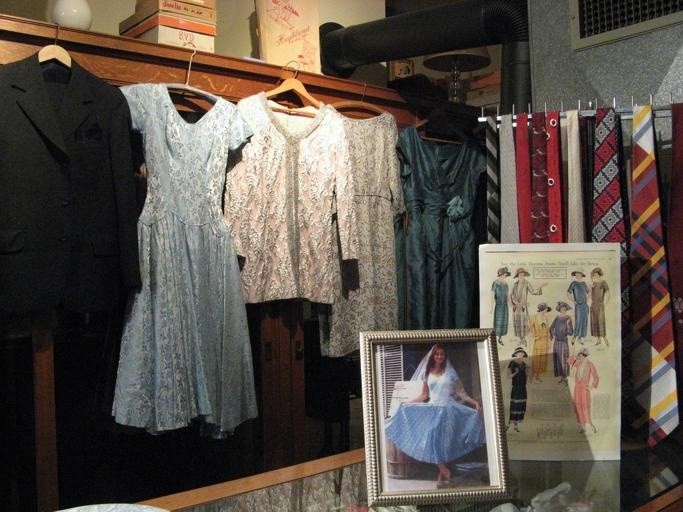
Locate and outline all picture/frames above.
[359,329,513,507]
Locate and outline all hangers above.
[415,98,466,144]
[265,60,321,117]
[165,42,219,103]
[37,22,72,68]
[332,82,385,115]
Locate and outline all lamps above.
[422,46,492,103]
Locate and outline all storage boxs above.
[119,0,217,54]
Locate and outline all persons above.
[502,347,528,433]
[588,267,610,347]
[509,267,547,345]
[528,301,551,384]
[384,344,487,488]
[566,267,590,344]
[490,266,510,346]
[549,301,573,385]
[565,349,598,433]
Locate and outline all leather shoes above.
[435,475,453,489]
